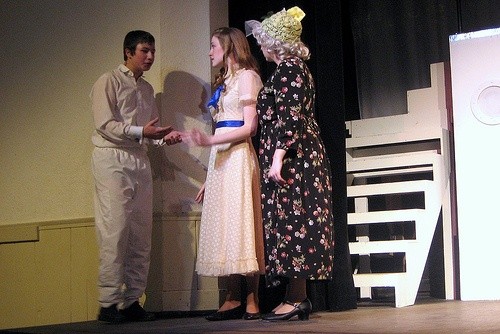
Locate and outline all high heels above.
[262,297,312,320]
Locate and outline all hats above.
[245,6,312,61]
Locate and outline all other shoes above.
[98,303,126,324]
[206,303,244,320]
[245,310,262,320]
[120,301,155,322]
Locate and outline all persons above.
[239,6,336,324]
[86,29,185,325]
[177,26,264,322]
